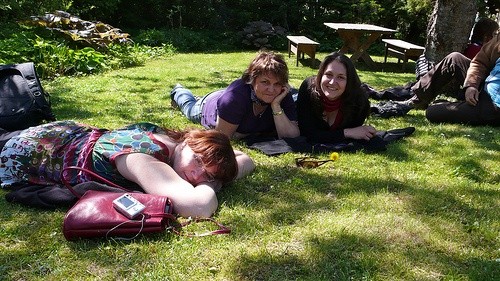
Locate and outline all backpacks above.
[0,62,55,133]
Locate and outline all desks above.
[323,22,398,69]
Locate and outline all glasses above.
[293,156,334,170]
[192,154,215,183]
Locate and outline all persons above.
[385,17,500,129]
[0,121,256,221]
[171,51,300,140]
[290,52,378,148]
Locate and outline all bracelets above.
[272,108,284,115]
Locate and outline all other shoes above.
[393,93,430,111]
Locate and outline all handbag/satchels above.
[60,165,175,241]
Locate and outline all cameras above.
[112,193,146,219]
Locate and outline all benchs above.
[286,35,321,67]
[381,39,425,65]
